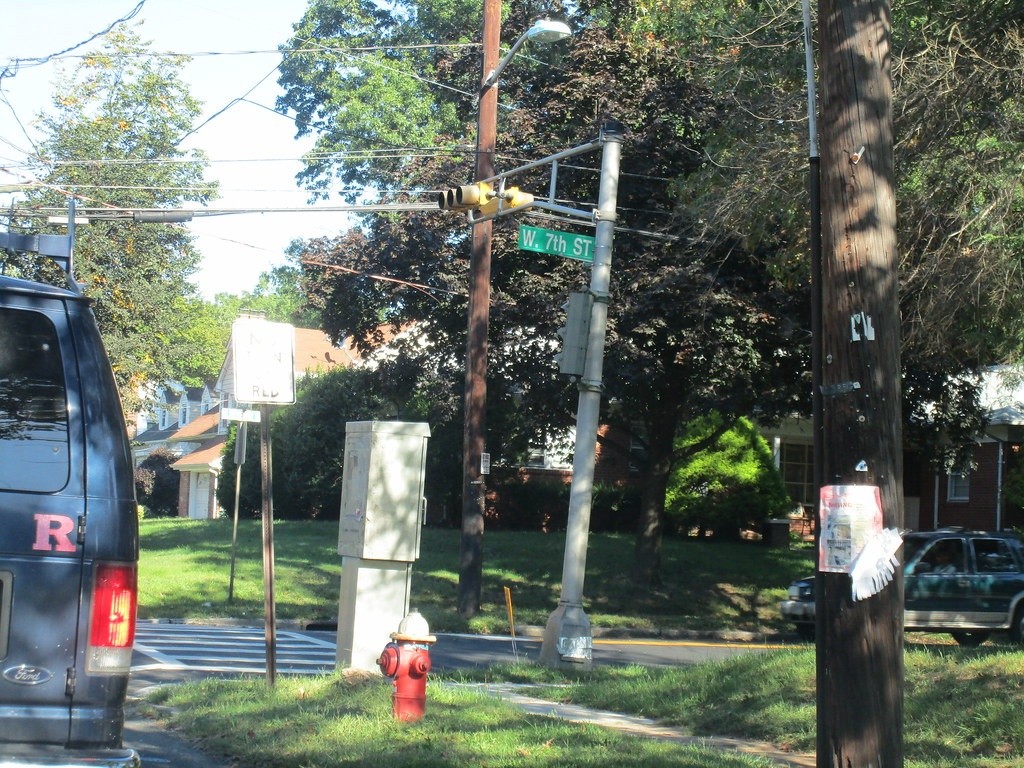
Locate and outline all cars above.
[783,524,1023,647]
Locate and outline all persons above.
[931,551,957,575]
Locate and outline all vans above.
[1,232,145,768]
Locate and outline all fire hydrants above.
[375,608,438,727]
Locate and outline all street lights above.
[466,1,570,625]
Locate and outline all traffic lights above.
[437,181,490,212]
